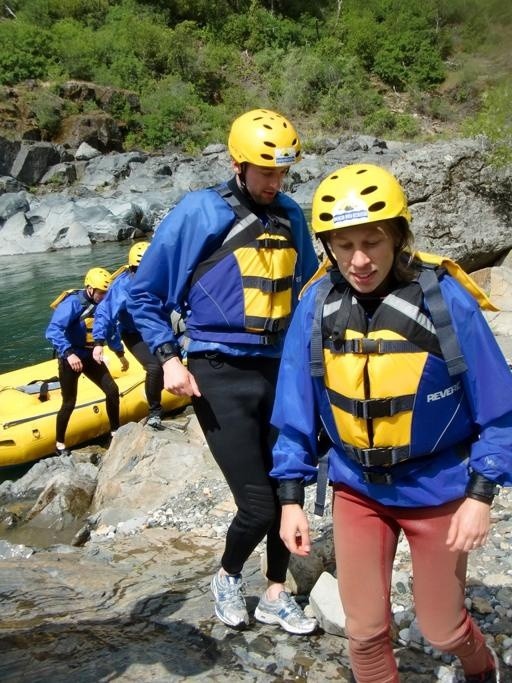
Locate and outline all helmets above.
[84,268,111,291]
[311,163,412,236]
[228,109,302,167]
[129,242,150,267]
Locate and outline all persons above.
[124,108,321,637]
[92,240,188,429]
[269,161,512,681]
[45,266,129,456]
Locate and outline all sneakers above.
[254,592,318,634]
[55,446,70,456]
[147,416,161,428]
[211,567,249,628]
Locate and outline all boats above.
[0,338,197,472]
